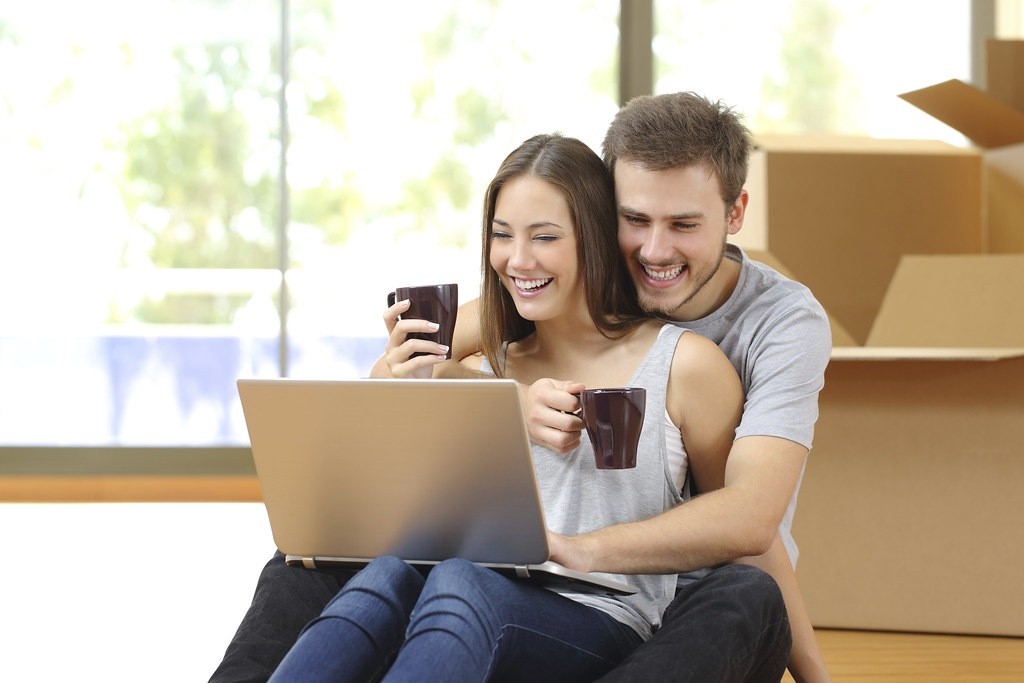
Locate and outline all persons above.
[208,91,831,683]
[265,133,831,683]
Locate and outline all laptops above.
[236,379,642,597]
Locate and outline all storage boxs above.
[726,137,982,345]
[739,249,1024,638]
[897,37,1024,257]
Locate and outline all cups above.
[566,388,646,470]
[387,284,458,362]
